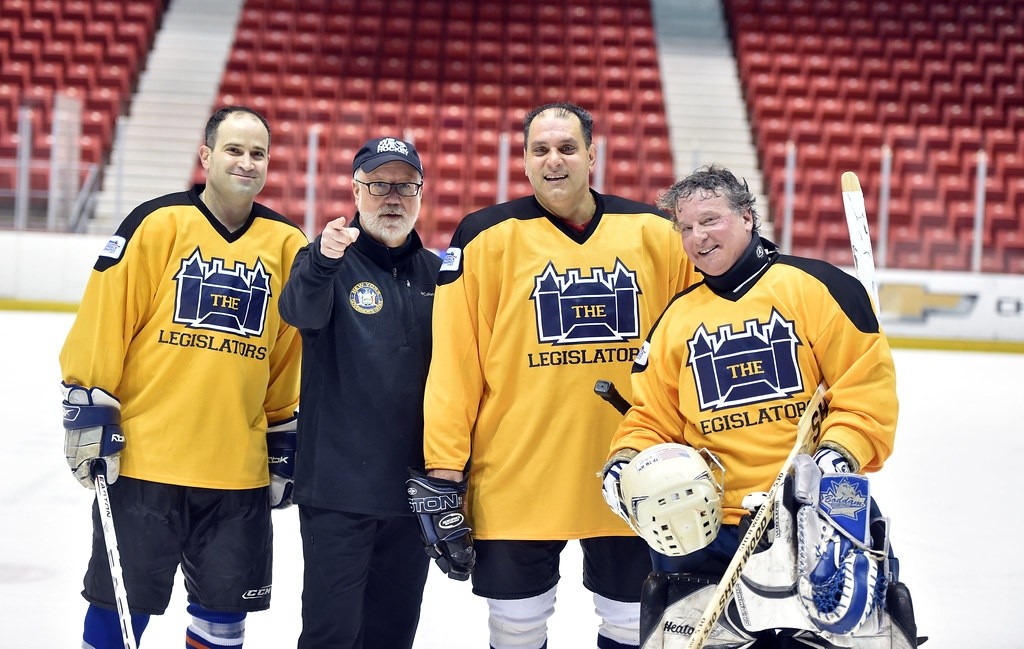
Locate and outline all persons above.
[59,105,927,649]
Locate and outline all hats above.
[352,137,423,180]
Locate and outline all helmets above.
[620,444,723,556]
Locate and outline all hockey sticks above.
[686,171,881,649]
[91,458,142,649]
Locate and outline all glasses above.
[354,178,423,197]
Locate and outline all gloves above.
[406,467,476,581]
[600,461,636,530]
[264,411,301,510]
[812,447,851,479]
[58,379,126,491]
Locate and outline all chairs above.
[195,0,678,249]
[0,0,172,230]
[722,0,1024,275]
